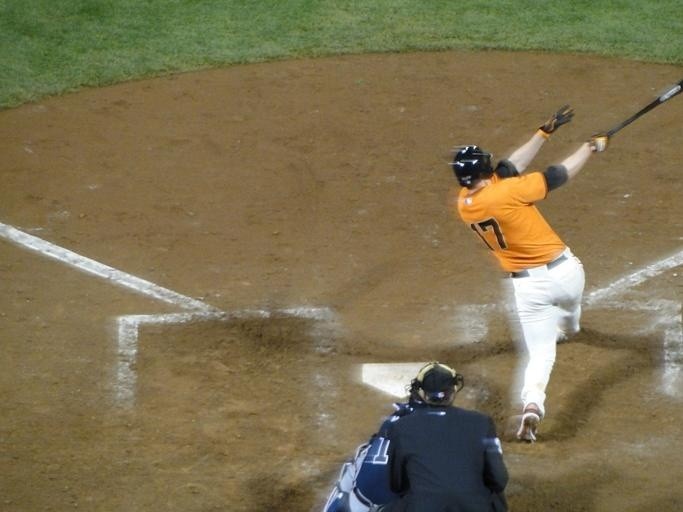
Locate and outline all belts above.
[353,486,371,507]
[511,256,567,278]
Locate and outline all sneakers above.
[516,403,540,441]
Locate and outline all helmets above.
[452,144,492,186]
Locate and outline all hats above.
[416,361,455,391]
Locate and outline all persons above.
[388,362,509,511]
[324,388,418,511]
[452,104,609,442]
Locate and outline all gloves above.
[586,133,608,152]
[538,104,574,139]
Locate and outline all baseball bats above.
[607,80,683,137]
